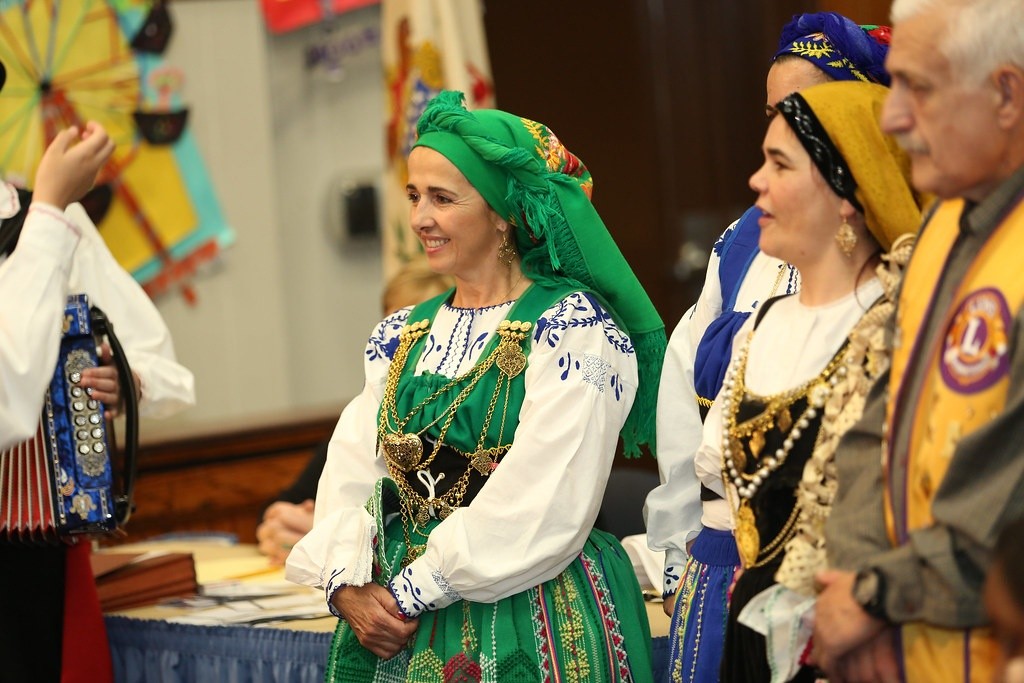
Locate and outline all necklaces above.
[495,273,526,305]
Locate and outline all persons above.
[648,1,1024,683]
[253,261,460,563]
[0,61,196,683]
[315,89,653,683]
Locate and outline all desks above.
[90,540,673,683]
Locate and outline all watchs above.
[851,566,888,624]
